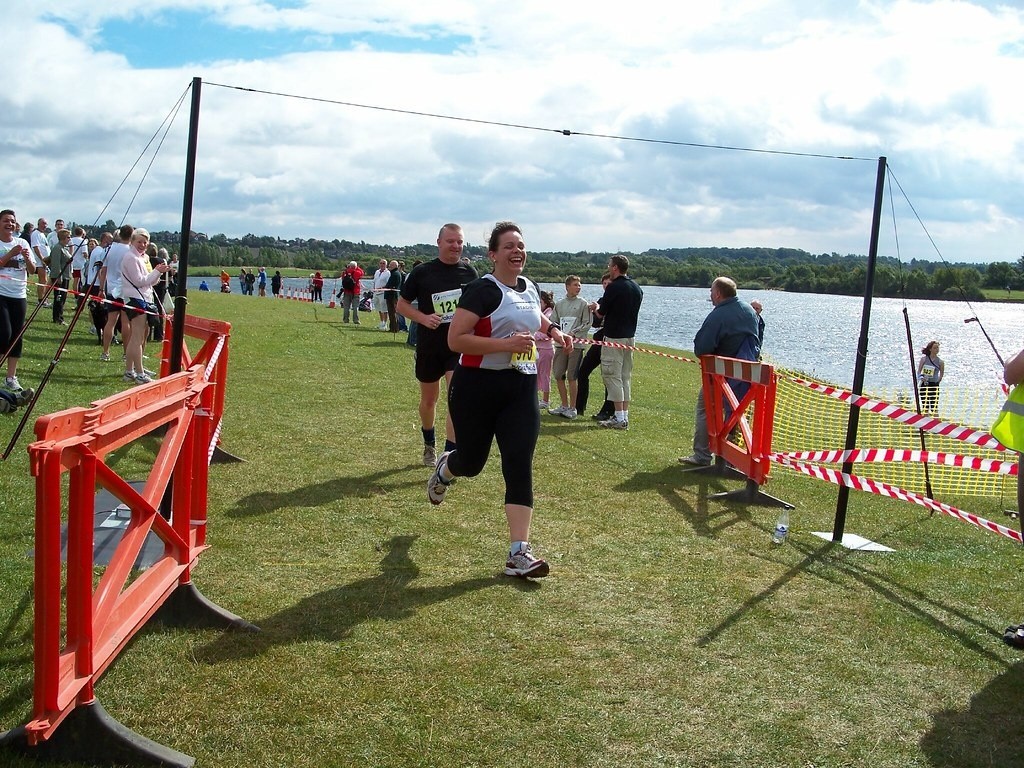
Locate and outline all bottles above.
[772,505,790,543]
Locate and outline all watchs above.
[547,322,561,338]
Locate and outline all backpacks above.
[342,270,356,290]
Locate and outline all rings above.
[529,341,534,347]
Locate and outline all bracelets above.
[0,259,7,265]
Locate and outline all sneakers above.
[548,405,569,415]
[562,408,577,418]
[134,366,156,376]
[135,374,154,384]
[423,441,438,467]
[123,371,137,381]
[427,451,457,505]
[4,376,23,391]
[596,414,629,429]
[505,542,549,578]
[100,352,110,362]
[123,354,126,362]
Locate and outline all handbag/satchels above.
[147,305,160,327]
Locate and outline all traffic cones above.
[293,288,297,300]
[286,286,292,299]
[326,288,337,309]
[279,285,284,298]
[302,289,308,302]
[307,288,313,302]
[298,288,303,301]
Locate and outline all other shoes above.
[379,324,388,331]
[577,408,584,416]
[592,408,615,420]
[1003,624,1024,648]
[678,457,699,465]
[539,399,550,409]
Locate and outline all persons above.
[678,278,765,465]
[219,270,231,293]
[271,271,282,298]
[395,223,479,467]
[917,341,944,434]
[372,257,470,346]
[309,271,323,303]
[576,255,643,430]
[0,210,35,413]
[533,290,555,409]
[548,275,593,419]
[342,261,373,324]
[239,267,266,297]
[427,220,574,579]
[13,218,179,384]
[990,350,1024,647]
[199,280,211,291]
